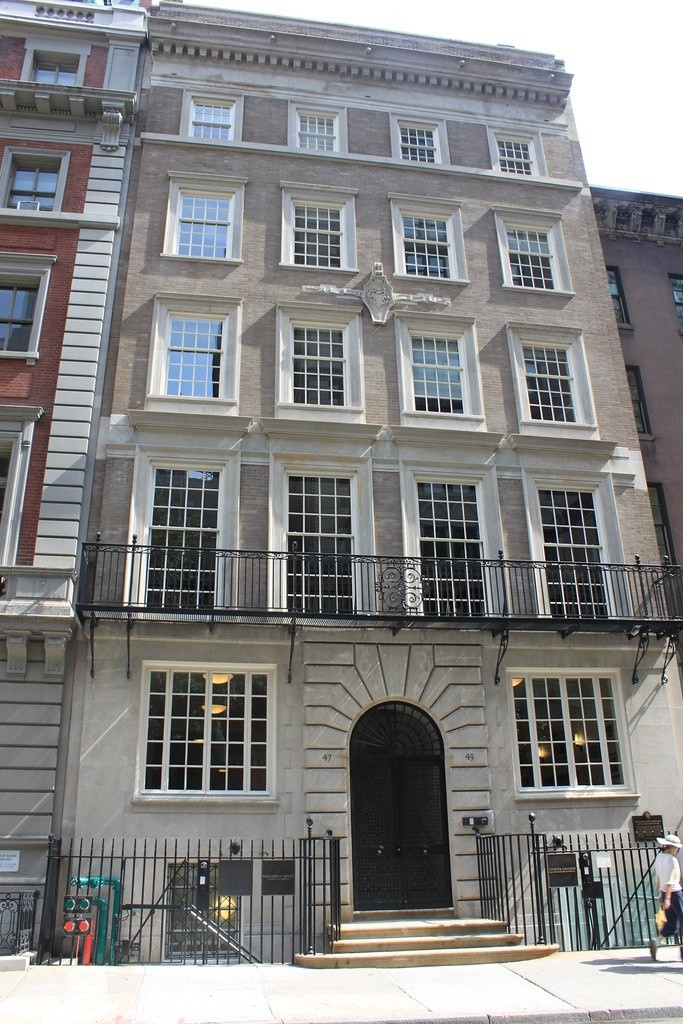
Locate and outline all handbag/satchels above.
[654,906,667,931]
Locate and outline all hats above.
[656,834,682,848]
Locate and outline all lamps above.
[202,705,226,714]
[202,673,233,684]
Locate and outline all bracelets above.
[665,898,671,900]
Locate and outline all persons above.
[649,835,683,961]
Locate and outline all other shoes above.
[680,946,683,960]
[647,940,657,960]
